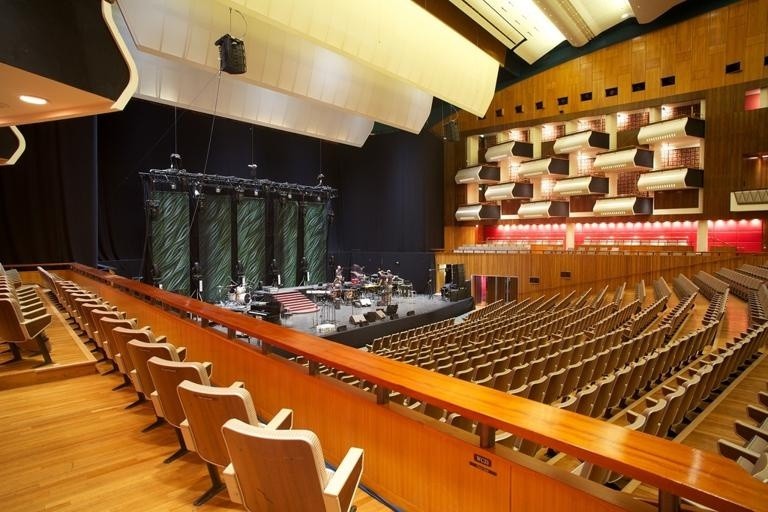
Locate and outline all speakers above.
[445,263,471,302]
[350,314,367,324]
[386,305,398,315]
[446,121,461,143]
[376,309,386,319]
[221,36,247,75]
[364,312,376,322]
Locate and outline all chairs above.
[145,355,244,465]
[176,380,295,505]
[111,324,188,409]
[291,261,763,512]
[37,266,151,377]
[455,242,737,257]
[1,263,54,369]
[123,337,214,434]
[223,417,366,512]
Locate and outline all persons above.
[324,264,394,304]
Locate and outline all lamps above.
[152,171,337,203]
[442,119,461,144]
[214,33,249,76]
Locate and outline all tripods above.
[185,288,204,323]
